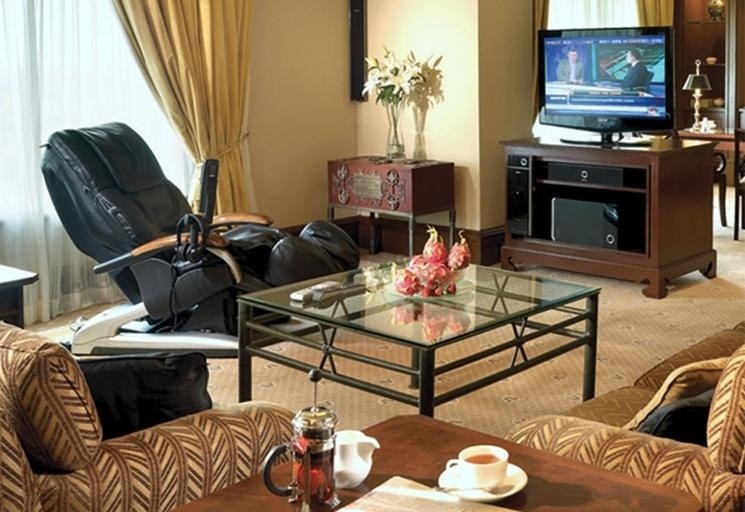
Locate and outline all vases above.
[384,97,405,159]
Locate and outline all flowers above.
[361,46,427,145]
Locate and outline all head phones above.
[175,213,209,263]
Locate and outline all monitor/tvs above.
[538,25,675,148]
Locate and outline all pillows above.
[627,355,731,434]
[3,329,213,473]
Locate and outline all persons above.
[617,49,648,87]
[556,48,586,85]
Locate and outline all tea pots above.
[329,429,381,488]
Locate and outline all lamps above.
[682,74,712,131]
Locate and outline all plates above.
[384,280,476,300]
[438,463,528,502]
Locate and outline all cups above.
[445,446,511,492]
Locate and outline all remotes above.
[312,283,365,301]
[289,280,341,302]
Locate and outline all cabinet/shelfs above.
[672,0,736,135]
[500,147,719,299]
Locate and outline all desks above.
[0,264,40,324]
[166,413,704,512]
[326,155,456,258]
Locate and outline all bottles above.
[263,405,341,509]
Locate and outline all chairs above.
[733,128,745,241]
[40,121,361,358]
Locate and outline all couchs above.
[509,319,743,500]
[1,323,297,511]
